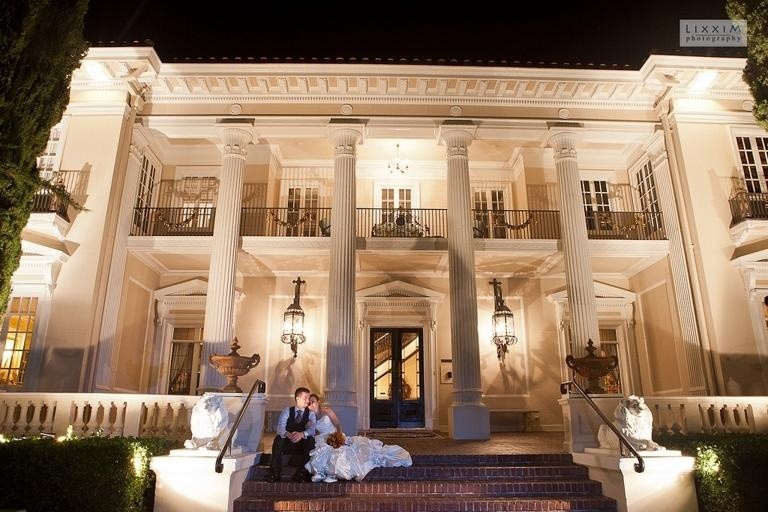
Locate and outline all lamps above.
[280,276,309,356]
[489,280,517,364]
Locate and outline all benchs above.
[488,408,539,432]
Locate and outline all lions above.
[597,394,666,451]
[183,391,239,452]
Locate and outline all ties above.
[295,410,302,425]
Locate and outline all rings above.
[297,439,298,441]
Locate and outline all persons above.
[304,394,413,483]
[266,388,317,484]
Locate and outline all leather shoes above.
[267,472,279,482]
[311,472,338,483]
[293,472,305,483]
